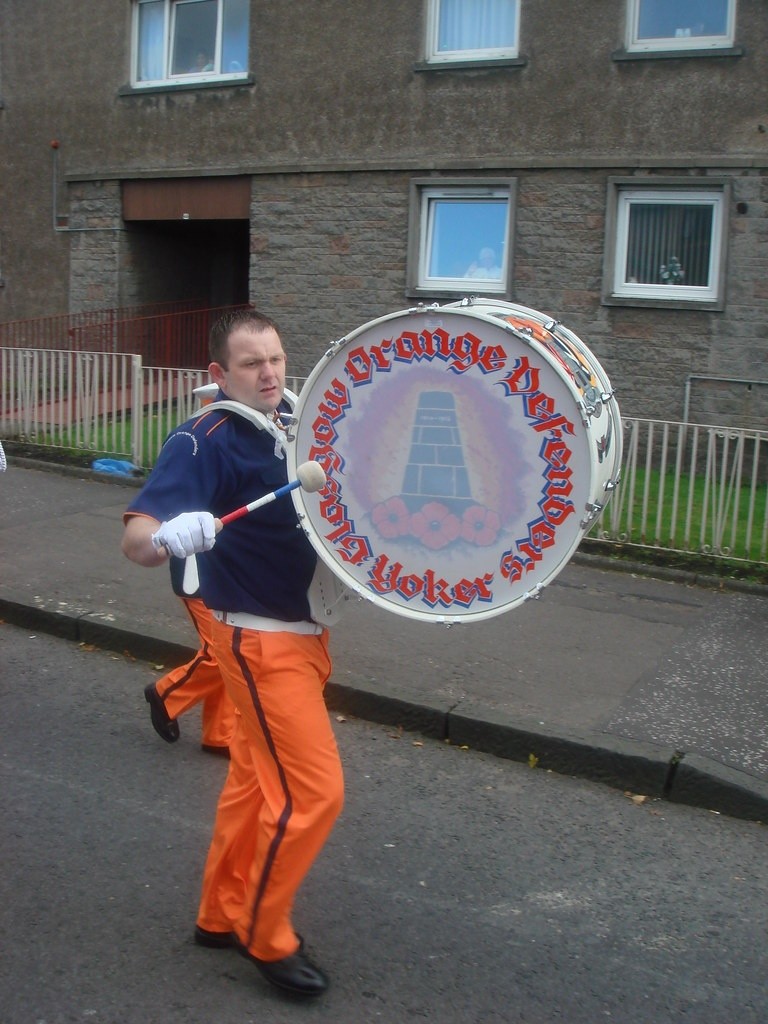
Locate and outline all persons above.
[122,308,343,998]
[144,384,296,764]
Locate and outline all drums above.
[290,296,624,622]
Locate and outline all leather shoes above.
[194,924,240,948]
[238,934,328,994]
[144,682,180,742]
[202,742,231,760]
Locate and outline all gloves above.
[150,510,216,557]
